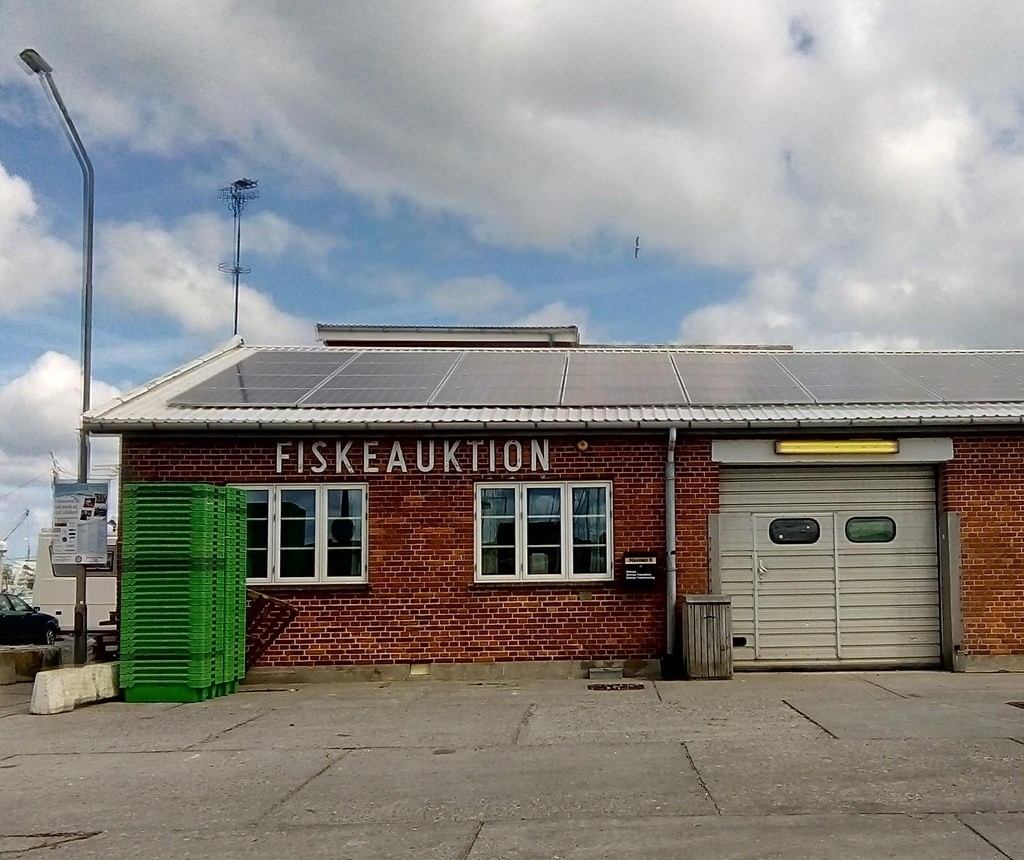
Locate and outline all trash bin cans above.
[681,592,737,681]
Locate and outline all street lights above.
[15,45,94,669]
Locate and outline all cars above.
[0,593,61,647]
